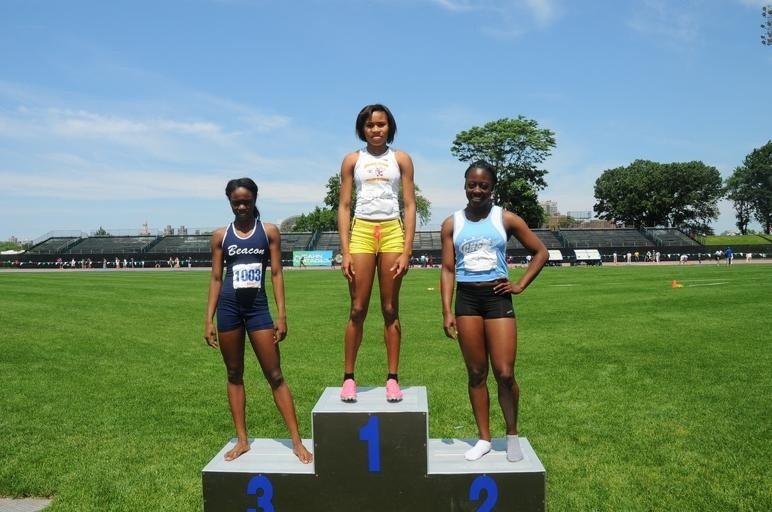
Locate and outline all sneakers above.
[340,378,357,403]
[386,379,404,403]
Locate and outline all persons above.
[439,160,549,461]
[204,178,314,464]
[680,255,687,263]
[410,254,433,268]
[713,248,723,265]
[337,104,416,402]
[626,251,631,263]
[725,247,732,265]
[656,252,660,262]
[300,257,305,268]
[612,251,617,262]
[634,250,639,262]
[329,256,336,270]
